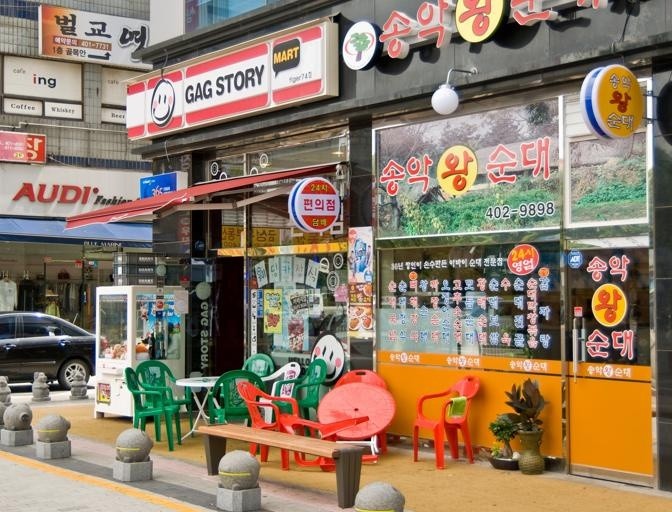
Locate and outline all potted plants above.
[480,377,561,475]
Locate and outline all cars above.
[0,311,108,391]
[305,291,347,320]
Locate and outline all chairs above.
[208,370,265,426]
[273,359,327,437]
[280,413,379,473]
[136,361,195,442]
[242,354,275,377]
[237,381,305,471]
[244,361,301,424]
[334,367,389,456]
[125,367,182,451]
[413,374,482,469]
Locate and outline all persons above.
[45,298,60,318]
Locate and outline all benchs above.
[198,423,364,509]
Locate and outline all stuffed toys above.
[101,342,149,359]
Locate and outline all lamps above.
[432,67,478,116]
